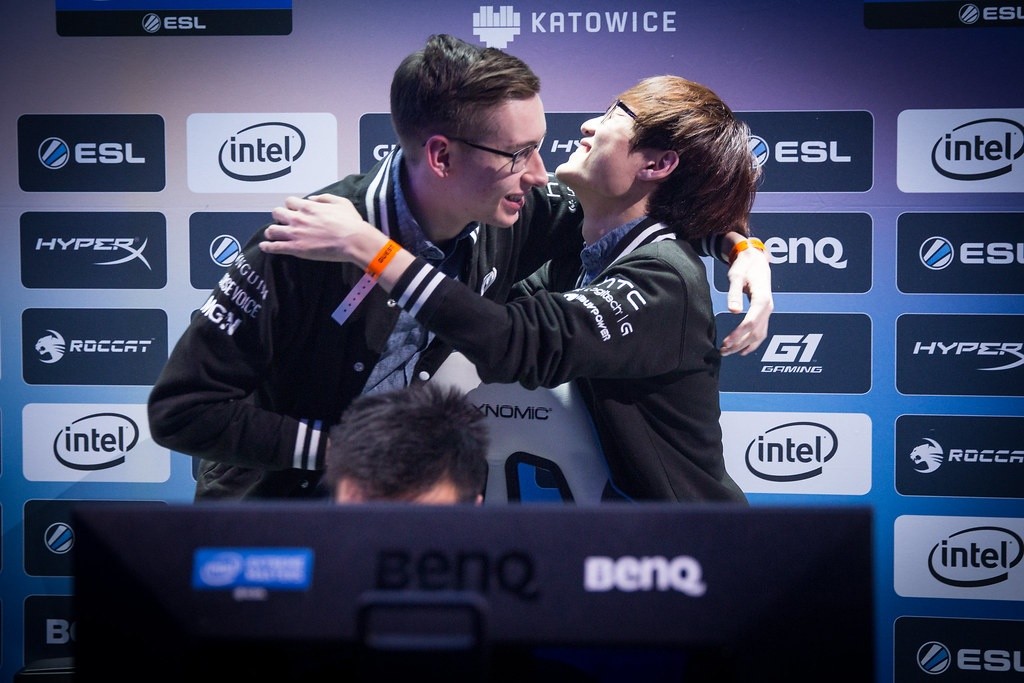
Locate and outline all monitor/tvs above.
[65,504,879,683]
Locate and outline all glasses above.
[421,134,544,173]
[599,98,640,126]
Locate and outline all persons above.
[258,74,764,507]
[326,380,489,506]
[150,34,773,503]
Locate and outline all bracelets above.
[729,236,766,265]
[332,239,403,326]
